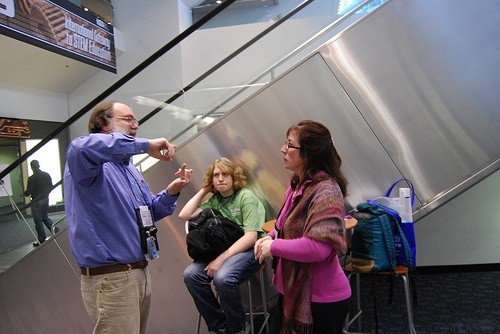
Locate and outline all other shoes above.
[33,241,40,246]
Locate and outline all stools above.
[195,259,270,334]
[343,265,417,334]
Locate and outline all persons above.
[62,101,194,334]
[178,157,268,334]
[23,160,60,248]
[254,119,351,334]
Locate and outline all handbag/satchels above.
[366,179,417,272]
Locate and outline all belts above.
[80,260,148,276]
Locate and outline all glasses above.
[112,116,139,128]
[282,141,302,151]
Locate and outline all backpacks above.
[186,208,244,262]
[350,203,414,274]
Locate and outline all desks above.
[261,217,358,249]
